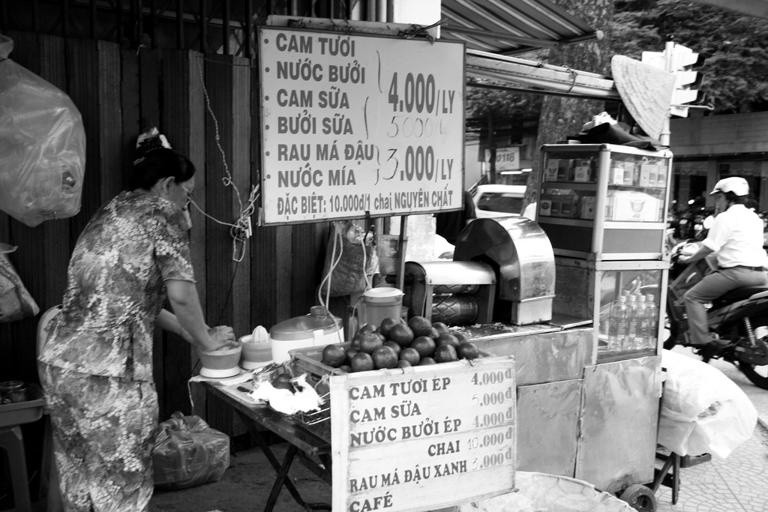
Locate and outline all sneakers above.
[702,342,718,362]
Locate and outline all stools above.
[0,426,32,512]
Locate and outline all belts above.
[738,264,766,271]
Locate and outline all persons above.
[677,175,767,356]
[430,190,476,245]
[36,148,237,512]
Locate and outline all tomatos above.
[323,315,479,371]
[273,374,291,389]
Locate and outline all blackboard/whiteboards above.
[328,355,519,512]
[257,26,466,226]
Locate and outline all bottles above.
[609,292,657,352]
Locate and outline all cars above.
[470,184,528,219]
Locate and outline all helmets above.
[710,176,749,196]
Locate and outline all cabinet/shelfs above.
[536,143,674,366]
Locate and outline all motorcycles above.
[664,237,768,392]
[667,198,768,248]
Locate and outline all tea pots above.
[361,286,406,331]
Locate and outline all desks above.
[202,376,332,512]
